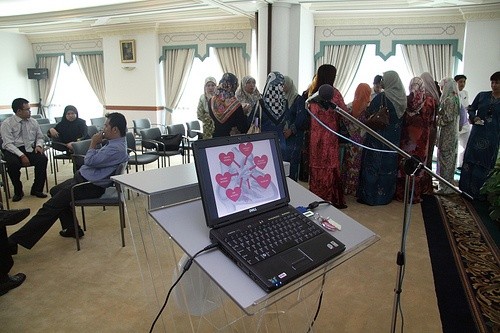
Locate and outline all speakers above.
[27,68,48,80]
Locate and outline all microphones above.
[305,84,336,104]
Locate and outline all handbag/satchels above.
[248,100,263,135]
[366,94,390,130]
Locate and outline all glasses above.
[19,108,31,112]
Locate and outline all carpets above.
[422,192,500,333]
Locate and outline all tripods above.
[37,80,46,118]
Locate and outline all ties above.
[20,120,34,153]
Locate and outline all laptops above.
[192,131,346,293]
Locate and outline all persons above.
[0,99,129,296]
[197,63,500,209]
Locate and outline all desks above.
[109,162,388,327]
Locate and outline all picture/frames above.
[119,39,136,62]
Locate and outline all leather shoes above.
[13,191,24,201]
[30,190,48,198]
[0,272,26,296]
[60,228,84,238]
[0,208,30,226]
[8,243,17,255]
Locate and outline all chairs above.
[0,114,204,252]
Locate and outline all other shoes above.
[334,204,348,209]
